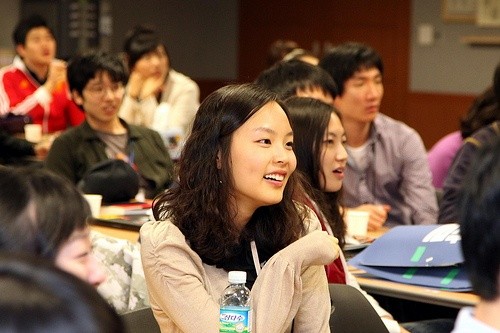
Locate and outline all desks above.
[87,199,479,308]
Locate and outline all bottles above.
[219,271,252,333]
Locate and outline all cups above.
[24,124,41,143]
[345,210,369,236]
[83,195,103,219]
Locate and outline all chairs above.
[119,284,390,333]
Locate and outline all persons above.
[283,96,411,333]
[139,83,340,333]
[0,259,125,333]
[0,166,107,291]
[451,134,500,333]
[0,16,500,244]
